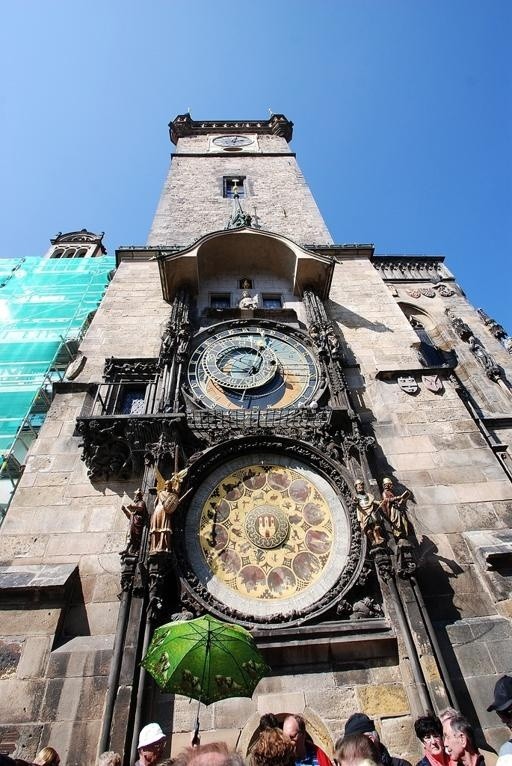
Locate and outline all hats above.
[137,722,167,749]
[343,718,376,738]
[486,674,512,712]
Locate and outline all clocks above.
[204,131,261,156]
[176,316,332,426]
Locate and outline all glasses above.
[495,708,512,719]
[147,740,167,751]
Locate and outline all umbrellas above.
[142,615,273,749]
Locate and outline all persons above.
[118,487,147,559]
[149,479,178,552]
[349,479,387,549]
[239,291,257,309]
[382,476,414,545]
